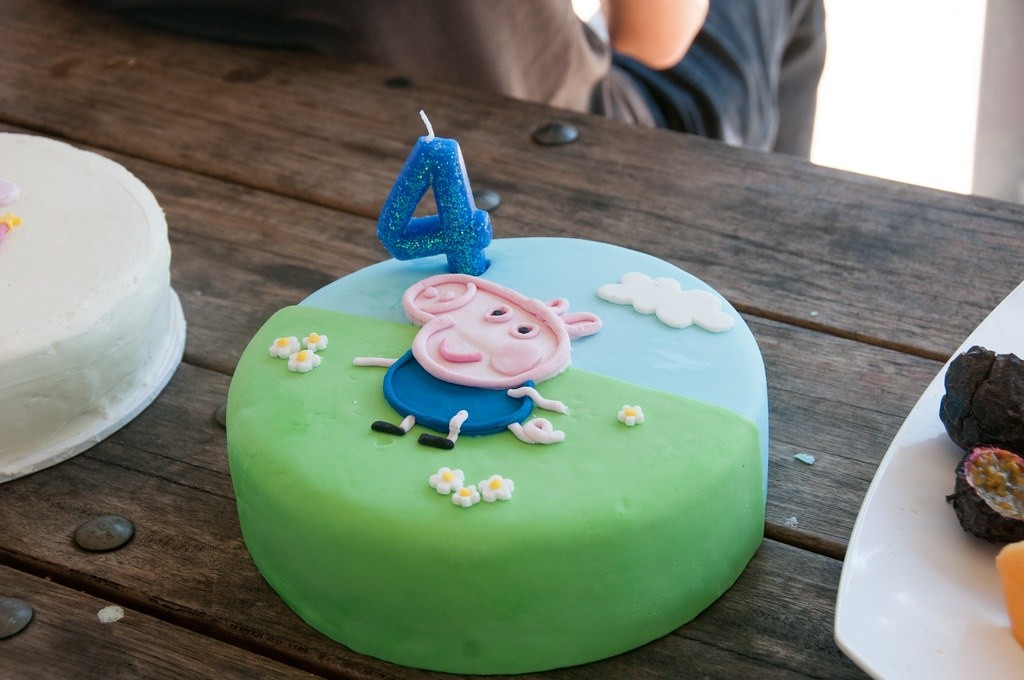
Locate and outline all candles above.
[375,108,494,276]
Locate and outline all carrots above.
[995,540,1024,653]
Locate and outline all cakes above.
[0,129,173,476]
[225,236,768,675]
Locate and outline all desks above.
[0,0,1024,680]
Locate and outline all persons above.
[53,0,821,157]
